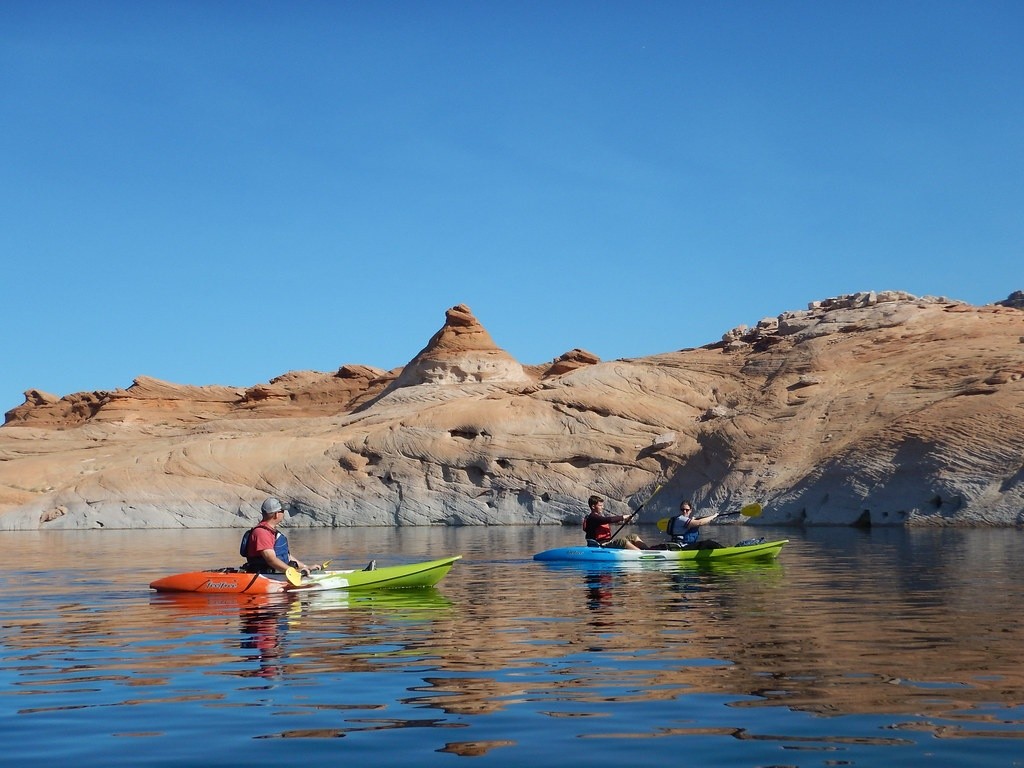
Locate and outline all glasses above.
[278,509,284,513]
[680,508,690,512]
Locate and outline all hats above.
[261,497,282,513]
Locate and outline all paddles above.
[285,557,336,587]
[597,484,661,546]
[658,501,763,532]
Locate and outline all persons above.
[246,496,376,575]
[671,500,724,550]
[583,495,650,550]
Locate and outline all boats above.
[532,539,789,562]
[149,554,465,594]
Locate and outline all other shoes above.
[362,560,376,571]
